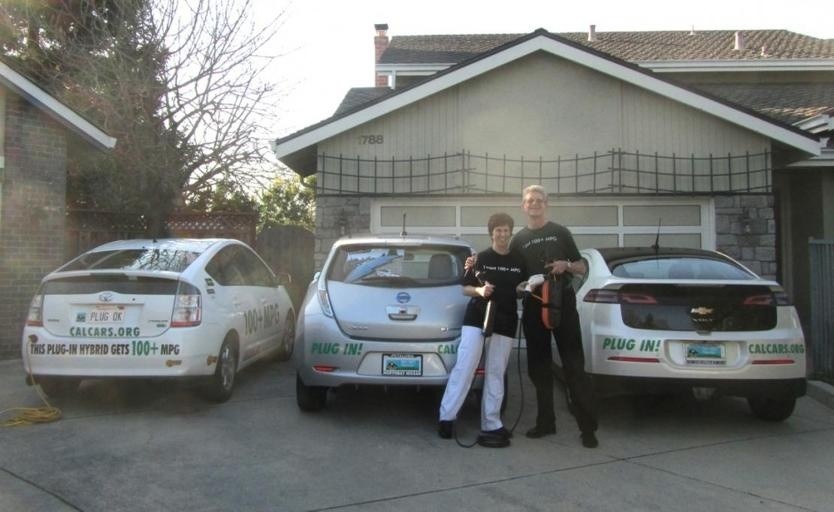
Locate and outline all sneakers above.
[439,421,453,439]
[579,431,598,448]
[527,424,556,438]
[482,427,513,438]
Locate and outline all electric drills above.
[523,274,552,306]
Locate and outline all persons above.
[509,184,601,447]
[436,210,530,440]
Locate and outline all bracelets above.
[567,261,572,272]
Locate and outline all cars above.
[545,217,809,423]
[291,213,510,421]
[16,234,296,403]
